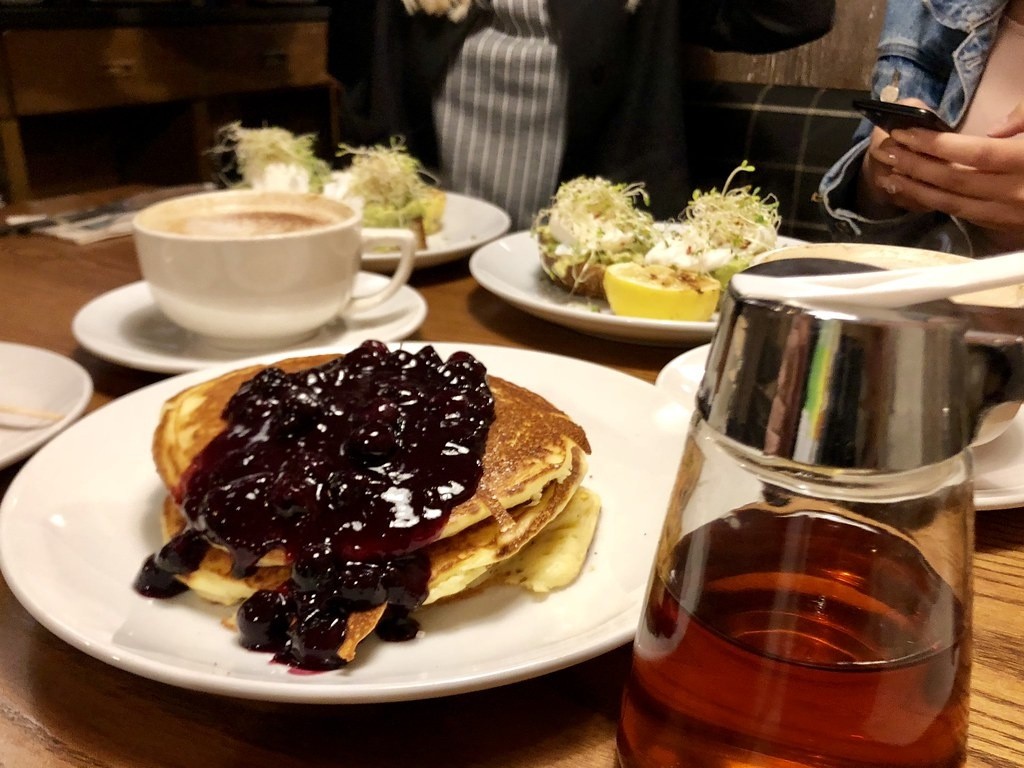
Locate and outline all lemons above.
[603,261,720,320]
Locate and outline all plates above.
[70,271,427,373]
[1,344,692,705]
[1,339,93,471]
[327,186,511,268]
[469,221,807,342]
[653,342,1024,511]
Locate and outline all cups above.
[131,190,416,345]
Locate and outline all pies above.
[152,353,602,662]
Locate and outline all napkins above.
[33,205,139,247]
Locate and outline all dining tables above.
[0,180,1024,768]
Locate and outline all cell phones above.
[849,97,956,135]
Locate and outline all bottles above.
[619,254,981,768]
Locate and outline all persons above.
[324,0,837,232]
[812,0,1024,254]
